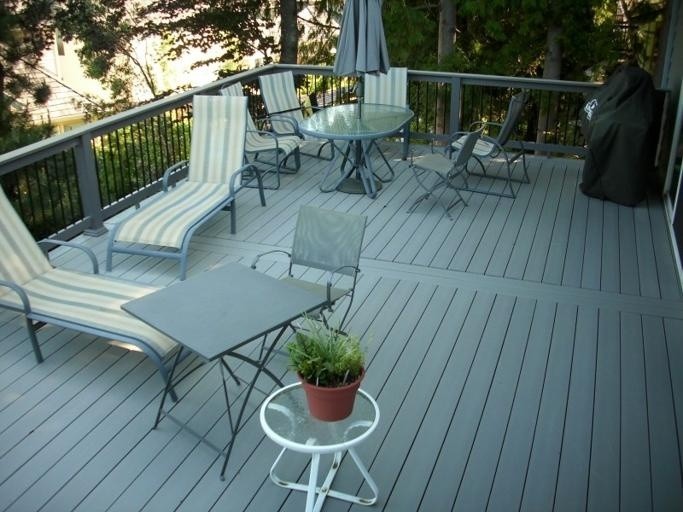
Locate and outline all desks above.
[259,380,381,511]
[119,259,326,482]
[298,102,415,200]
[119,258,381,512]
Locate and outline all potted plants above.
[278,309,376,424]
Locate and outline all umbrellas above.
[332,0,391,180]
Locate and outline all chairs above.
[362,69,410,163]
[450,89,531,199]
[252,203,370,365]
[220,82,305,191]
[402,121,486,220]
[107,93,267,282]
[257,71,336,170]
[1,187,185,402]
